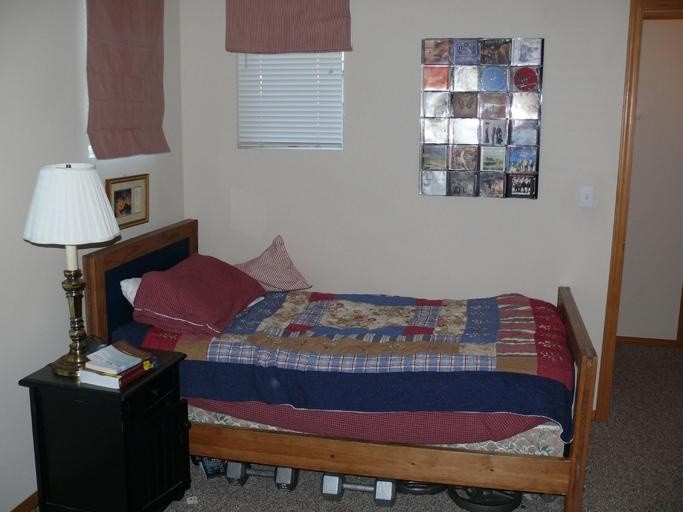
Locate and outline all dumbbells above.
[320,472,396,506]
[225,460,300,492]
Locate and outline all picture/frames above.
[105,172,150,229]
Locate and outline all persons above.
[114,191,132,218]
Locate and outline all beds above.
[80,217,599,511]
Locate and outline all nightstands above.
[17,346,192,512]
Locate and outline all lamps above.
[22,160,122,378]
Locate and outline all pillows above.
[120,277,142,306]
[233,234,312,293]
[133,253,267,337]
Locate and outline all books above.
[80,338,158,389]
